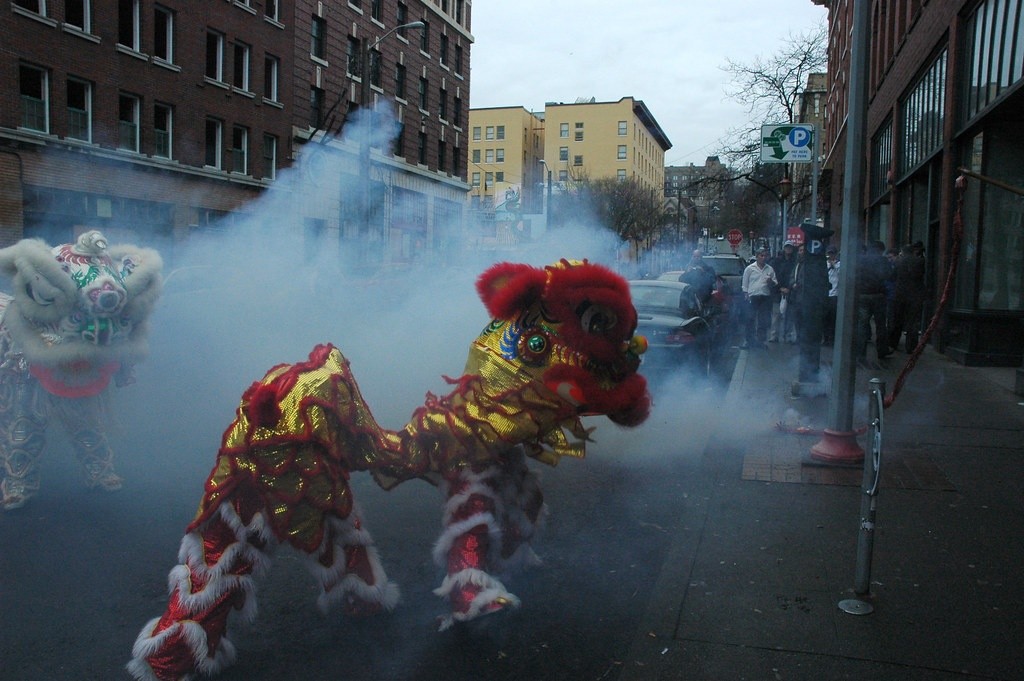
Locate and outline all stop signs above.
[728,230,743,245]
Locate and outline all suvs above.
[702,253,748,304]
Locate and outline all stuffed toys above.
[123,255,654,681]
[1,227,165,511]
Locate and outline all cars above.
[717,234,724,241]
[629,279,712,377]
[655,271,737,332]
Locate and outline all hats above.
[825,244,839,254]
[783,240,795,247]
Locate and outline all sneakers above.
[769,333,779,344]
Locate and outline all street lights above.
[355,21,425,286]
[538,159,552,232]
[779,178,791,245]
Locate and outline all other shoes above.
[740,336,769,349]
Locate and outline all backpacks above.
[855,260,879,293]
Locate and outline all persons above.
[687,238,936,367]
